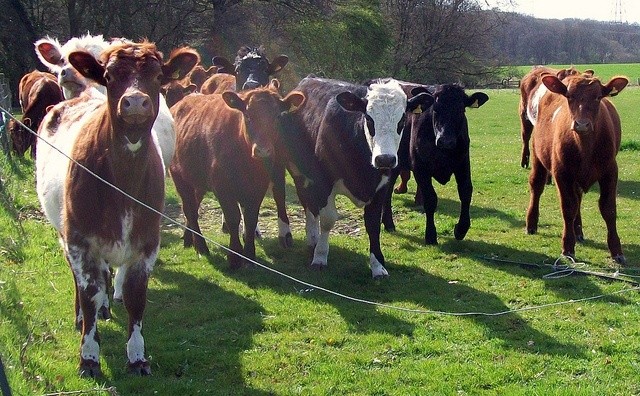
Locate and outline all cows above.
[360,80,489,244]
[160,81,196,109]
[35,34,201,379]
[186,66,217,92]
[526,74,629,266]
[4,69,66,163]
[199,45,289,240]
[272,73,434,280]
[167,80,306,272]
[520,65,594,184]
[393,82,423,205]
[31,31,176,175]
